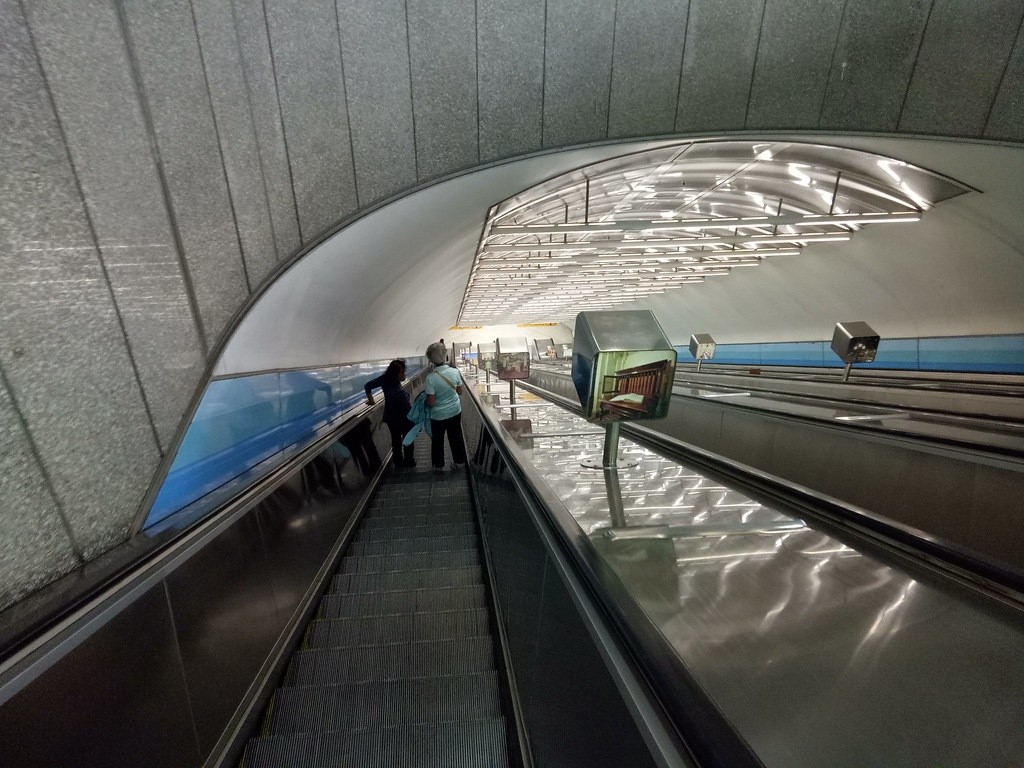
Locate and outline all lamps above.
[490,210,923,234]
[478,248,803,266]
[475,260,761,274]
[456,269,733,328]
[482,229,854,253]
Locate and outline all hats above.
[426,342,447,362]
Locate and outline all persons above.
[222,371,383,535]
[423,341,466,474]
[364,360,419,473]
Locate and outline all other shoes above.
[433,466,445,477]
[451,464,461,472]
